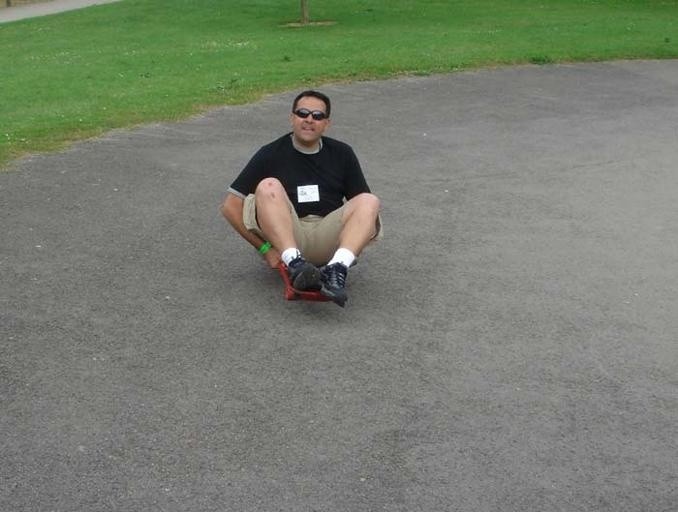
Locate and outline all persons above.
[221,90,383,305]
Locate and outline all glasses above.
[293,107,328,120]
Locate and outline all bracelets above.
[257,240,272,256]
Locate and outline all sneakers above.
[286,256,348,309]
